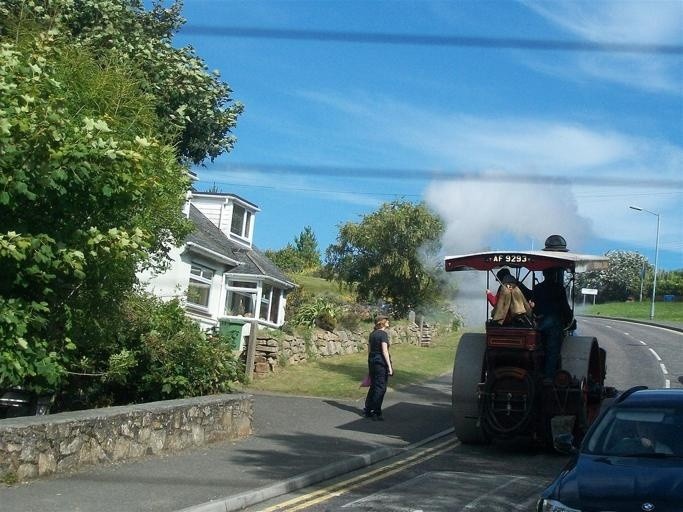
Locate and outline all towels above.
[218,317,244,350]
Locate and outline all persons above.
[517,236,577,389]
[620,417,674,455]
[485,267,536,328]
[364,315,394,422]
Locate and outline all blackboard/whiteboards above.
[444,232,615,458]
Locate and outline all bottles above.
[374,315,388,324]
[542,235,570,252]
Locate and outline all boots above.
[536,385,683,512]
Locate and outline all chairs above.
[363,409,383,421]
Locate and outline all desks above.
[629,204,662,320]
